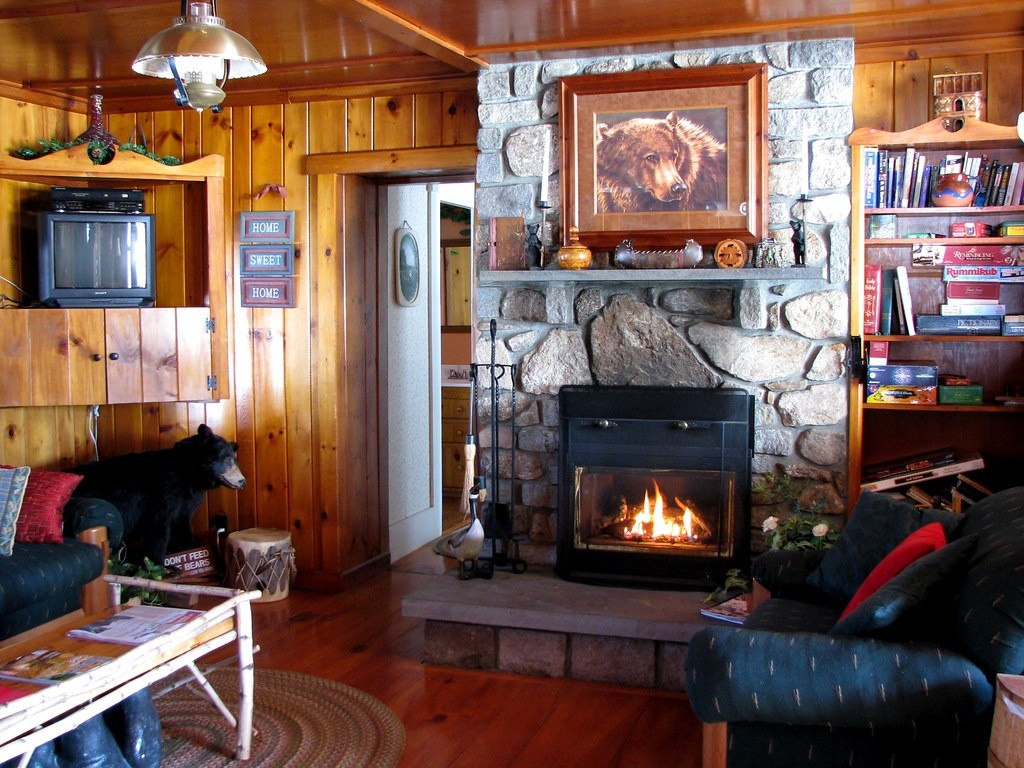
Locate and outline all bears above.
[62,424,248,579]
[595,111,728,216]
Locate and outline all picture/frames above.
[558,59,771,253]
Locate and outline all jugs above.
[930,173,985,207]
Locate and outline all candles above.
[540,126,551,201]
[799,118,809,196]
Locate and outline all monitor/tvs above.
[37,210,157,309]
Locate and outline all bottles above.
[74,94,122,161]
[558,226,592,270]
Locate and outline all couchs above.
[683,483,1024,768]
[0,462,128,650]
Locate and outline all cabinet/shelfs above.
[0,306,232,411]
[442,386,470,498]
[440,237,470,332]
[848,115,1024,511]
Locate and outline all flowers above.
[755,470,833,549]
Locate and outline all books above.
[864,265,916,336]
[915,266,1024,336]
[66,605,207,645]
[860,443,985,493]
[700,572,753,625]
[0,645,117,719]
[859,146,1024,207]
[911,244,1024,271]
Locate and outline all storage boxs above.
[939,384,984,405]
[866,359,939,385]
[869,341,889,364]
[865,384,939,406]
[941,373,971,387]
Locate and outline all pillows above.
[803,487,966,597]
[842,518,947,620]
[0,466,32,557]
[831,528,981,633]
[0,465,87,544]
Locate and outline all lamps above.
[130,0,270,115]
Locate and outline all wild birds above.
[446,483,484,579]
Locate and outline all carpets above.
[149,664,411,768]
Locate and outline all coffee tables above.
[0,577,263,768]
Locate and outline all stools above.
[229,530,294,602]
